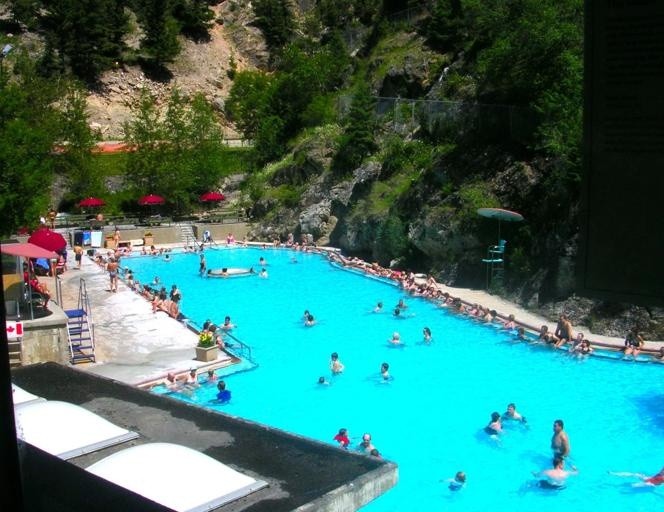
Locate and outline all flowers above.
[196,331,217,348]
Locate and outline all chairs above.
[482,240,507,263]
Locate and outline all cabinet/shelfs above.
[24,286,46,318]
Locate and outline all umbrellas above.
[1,242,60,319]
[79,197,106,214]
[200,191,224,210]
[29,229,67,253]
[137,194,165,219]
[477,206,525,244]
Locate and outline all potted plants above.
[144,231,154,245]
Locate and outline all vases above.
[195,345,219,362]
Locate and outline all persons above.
[73,242,83,269]
[96,212,103,222]
[39,215,46,226]
[49,209,56,229]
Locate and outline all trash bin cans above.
[74,232,83,246]
